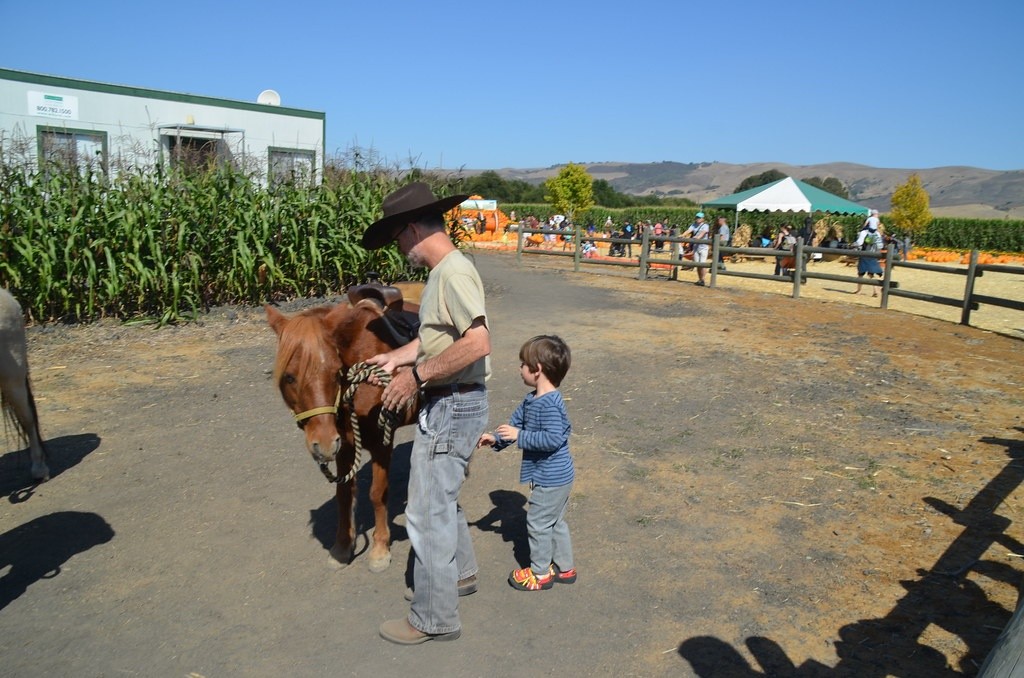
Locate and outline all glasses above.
[386,220,417,249]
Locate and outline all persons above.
[509,209,730,286]
[476,334,577,591]
[902,233,911,262]
[849,210,883,298]
[477,211,486,233]
[362,183,492,644]
[754,217,839,276]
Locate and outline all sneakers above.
[404,574,478,601]
[379,617,462,645]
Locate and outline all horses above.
[262,280,426,575]
[0,286,51,484]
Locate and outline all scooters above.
[609,230,626,258]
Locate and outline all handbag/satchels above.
[689,242,693,251]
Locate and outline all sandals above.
[509,567,554,591]
[550,563,577,584]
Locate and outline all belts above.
[423,382,482,396]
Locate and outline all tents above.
[698,176,878,234]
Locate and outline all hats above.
[695,212,705,218]
[361,182,469,251]
[870,210,878,213]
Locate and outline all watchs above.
[410,365,430,391]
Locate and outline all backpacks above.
[782,232,796,250]
[866,230,884,252]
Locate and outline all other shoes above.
[855,292,862,295]
[695,281,705,285]
[873,294,878,297]
